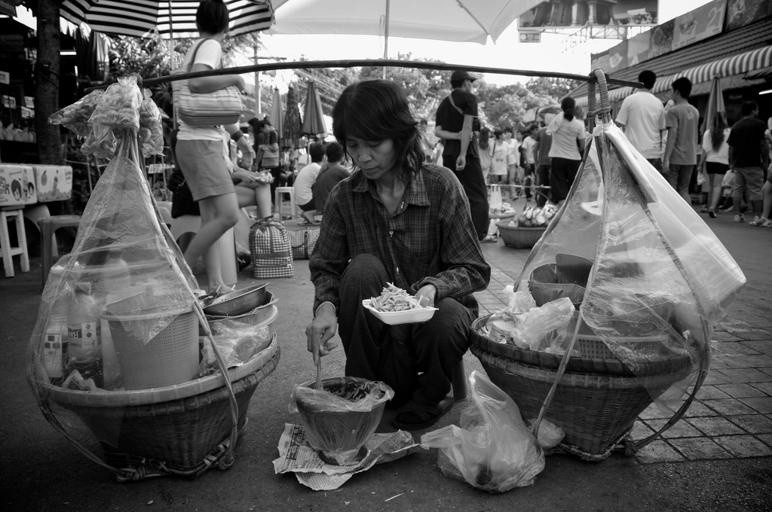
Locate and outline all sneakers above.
[734,213,772,227]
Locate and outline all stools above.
[0,205,32,279]
[35,213,89,287]
[273,187,297,222]
[169,215,202,244]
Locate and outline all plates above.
[361,298,440,326]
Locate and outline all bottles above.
[102,247,132,383]
[44,265,105,389]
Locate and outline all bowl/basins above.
[297,376,392,466]
[200,283,281,357]
[495,218,547,248]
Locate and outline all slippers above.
[390,397,456,431]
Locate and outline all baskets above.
[25,346,283,482]
[499,218,546,247]
[468,307,709,465]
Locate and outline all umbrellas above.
[281,83,305,145]
[262,0,550,79]
[59,0,275,72]
[302,79,328,142]
[269,86,284,145]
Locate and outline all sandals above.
[700,205,720,219]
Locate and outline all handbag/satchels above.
[177,74,243,126]
[287,227,322,261]
[248,215,296,279]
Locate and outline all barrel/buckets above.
[54,259,172,285]
[98,289,205,390]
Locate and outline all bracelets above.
[230,129,244,143]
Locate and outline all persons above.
[420,117,444,166]
[662,77,700,205]
[304,79,491,432]
[179,0,246,293]
[545,97,585,204]
[475,123,552,204]
[168,114,356,225]
[615,70,667,175]
[698,100,772,227]
